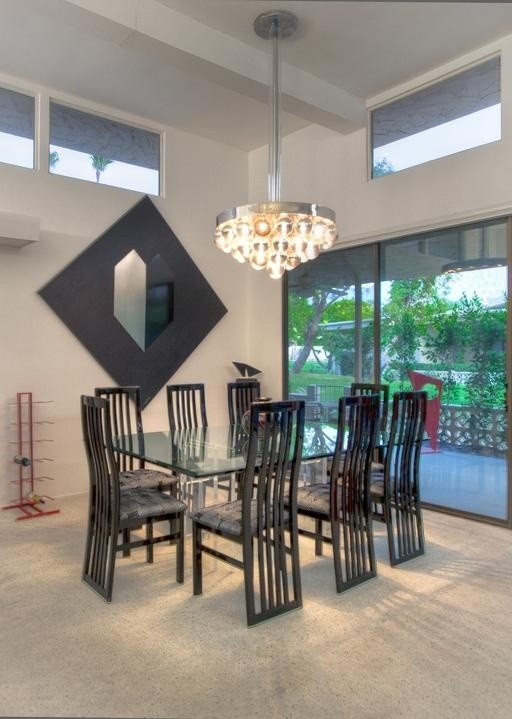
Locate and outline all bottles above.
[242,398,281,439]
[243,438,284,465]
[25,493,45,504]
[14,453,30,467]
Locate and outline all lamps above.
[214,8,338,283]
